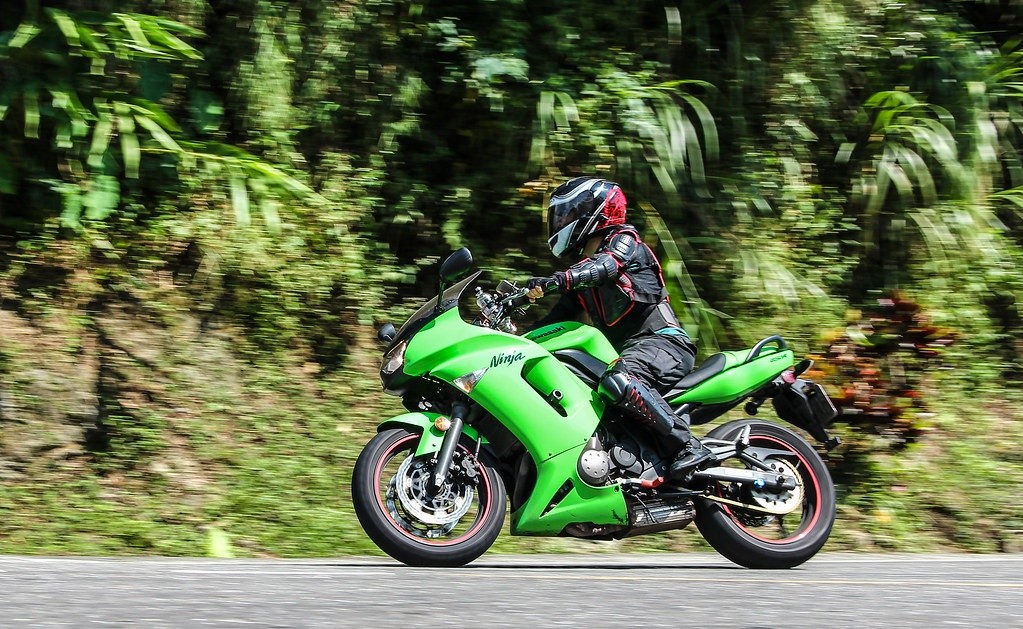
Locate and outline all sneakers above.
[670,435,717,476]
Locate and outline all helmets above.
[547,176,627,259]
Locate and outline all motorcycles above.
[352,247,841,568]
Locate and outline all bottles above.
[475,287,518,335]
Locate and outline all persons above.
[524,176,717,479]
